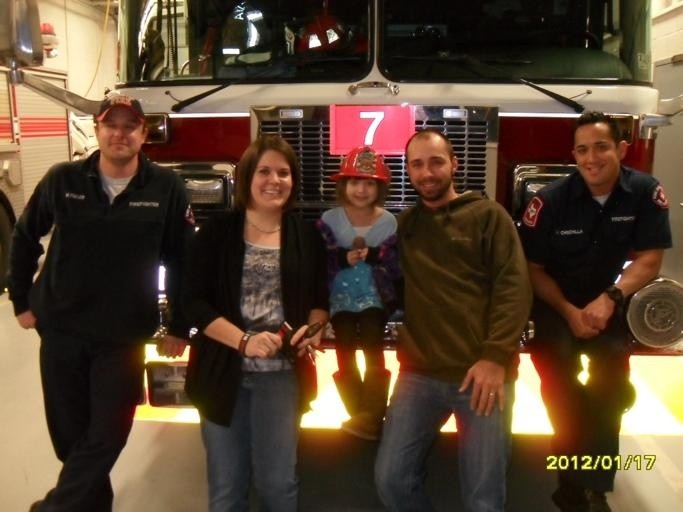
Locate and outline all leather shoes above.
[586,489,614,512]
[551,487,591,512]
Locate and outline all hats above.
[330,146,392,187]
[94,96,146,127]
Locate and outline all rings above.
[490,392,494,397]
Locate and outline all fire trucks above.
[1,1,114,297]
[95,0,680,407]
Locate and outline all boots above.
[331,364,392,441]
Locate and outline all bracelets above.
[239,333,256,359]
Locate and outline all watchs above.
[604,285,625,311]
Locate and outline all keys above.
[305,343,325,366]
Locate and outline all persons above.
[6,94,197,512]
[373,130,534,512]
[185,134,330,511]
[518,109,672,512]
[321,146,398,450]
